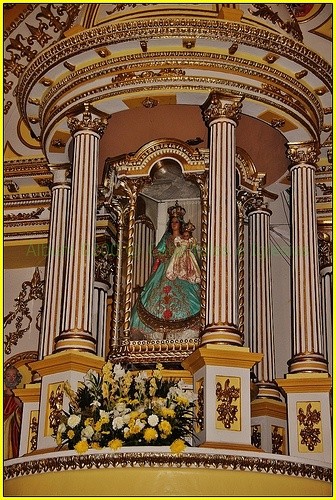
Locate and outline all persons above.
[4,366,24,461]
[130,199,200,340]
[166,230,202,284]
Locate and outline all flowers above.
[49,361,201,455]
